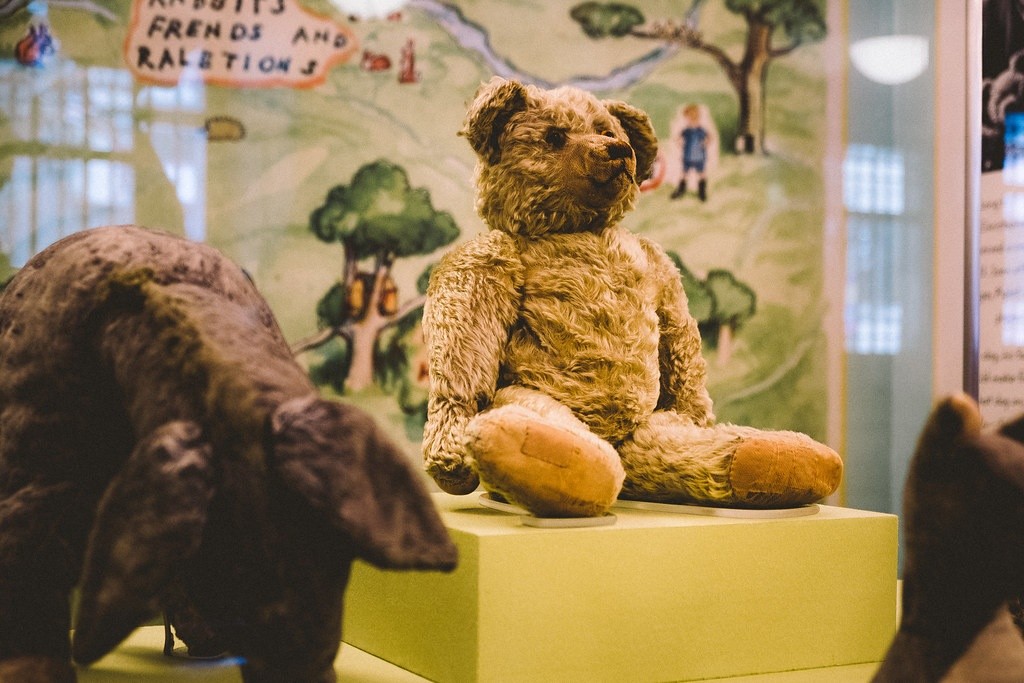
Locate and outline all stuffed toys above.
[421,75,844,519]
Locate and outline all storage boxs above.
[342,490,899,683]
